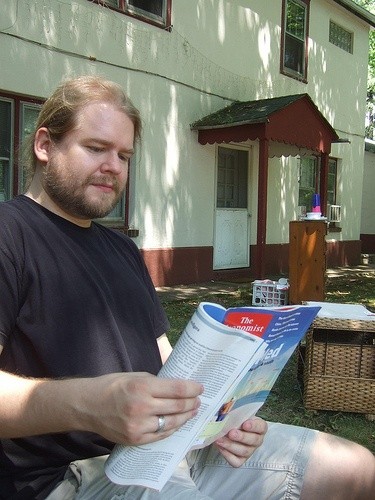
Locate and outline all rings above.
[155,416,166,432]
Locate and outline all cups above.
[330,205,341,222]
[296,206,306,220]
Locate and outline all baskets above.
[295,302,375,421]
[251,284,288,307]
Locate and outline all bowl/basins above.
[306,212,322,219]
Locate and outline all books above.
[104,302,322,492]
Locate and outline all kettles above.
[312,194,321,212]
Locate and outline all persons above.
[0,75,375,500]
